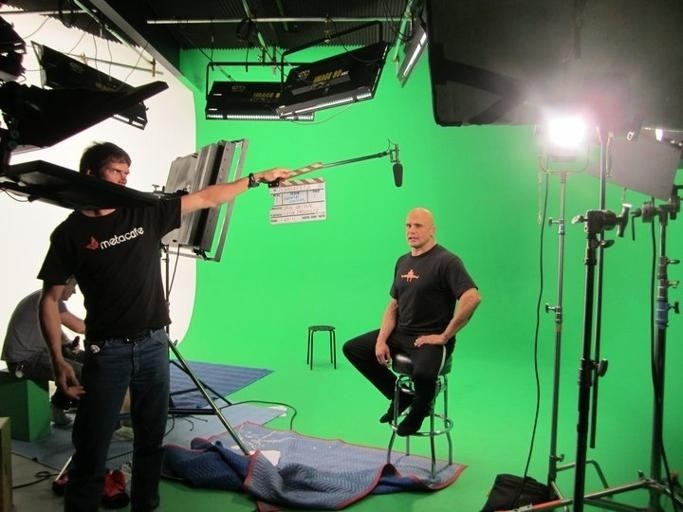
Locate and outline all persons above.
[38,143,291,511]
[342,208,483,436]
[0,275,86,426]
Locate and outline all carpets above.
[11,399,286,474]
[168,359,274,411]
[158,421,466,511]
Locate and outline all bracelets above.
[248,173,259,187]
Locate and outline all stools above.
[0,368,51,443]
[386,352,453,478]
[307,325,336,371]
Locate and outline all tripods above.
[512,197,682,511]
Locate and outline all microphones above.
[392,144,403,187]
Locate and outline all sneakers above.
[397,406,425,436]
[48,400,72,425]
[379,383,415,423]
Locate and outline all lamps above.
[541,99,579,164]
